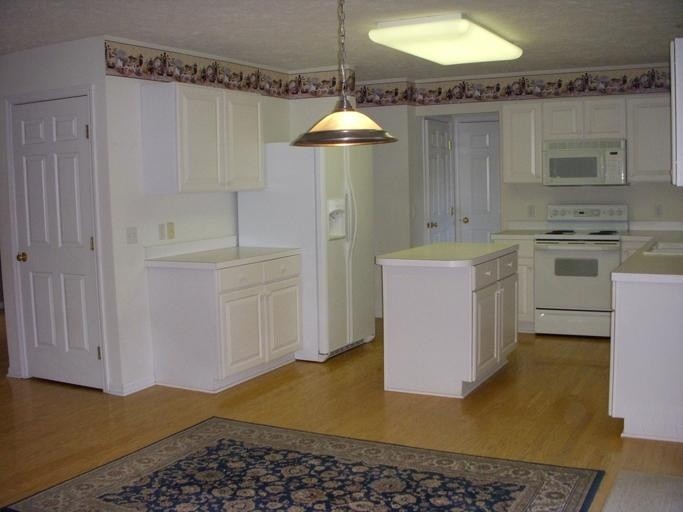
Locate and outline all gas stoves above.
[534,228,624,240]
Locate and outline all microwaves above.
[540,138,629,187]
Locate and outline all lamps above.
[286,1,525,151]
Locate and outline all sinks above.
[644,240,682,252]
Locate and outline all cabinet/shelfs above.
[381,246,520,381]
[140,82,265,198]
[148,252,303,395]
[609,239,682,422]
[501,93,671,184]
[493,239,534,324]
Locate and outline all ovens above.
[533,239,621,339]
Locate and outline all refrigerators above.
[235,137,377,364]
